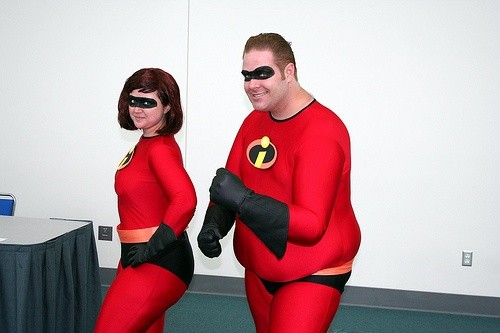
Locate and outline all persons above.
[197,33,361,333]
[93,69,198,333]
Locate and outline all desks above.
[0,215,103,333]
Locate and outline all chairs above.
[0,192,16,216]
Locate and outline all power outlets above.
[98,226,112,241]
[462,251,472,266]
